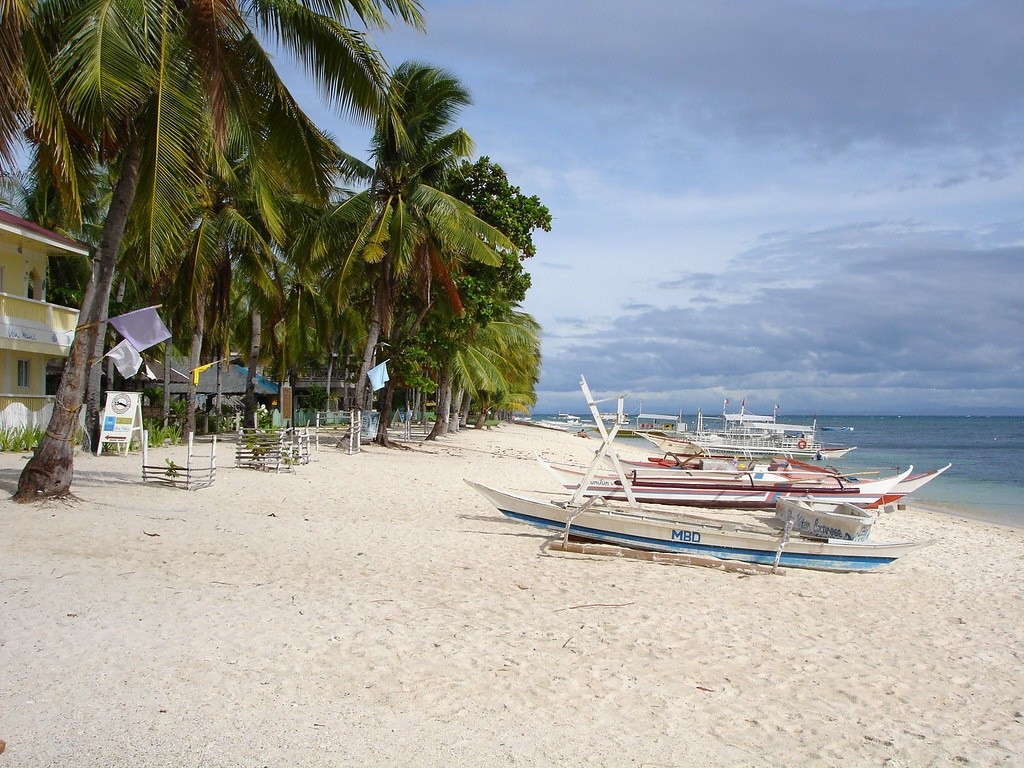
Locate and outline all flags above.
[367,362,389,391]
[105,339,143,380]
[194,364,211,387]
[106,306,172,353]
[142,365,157,380]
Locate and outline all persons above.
[582,429,591,438]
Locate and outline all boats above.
[530,390,952,535]
[460,477,937,575]
